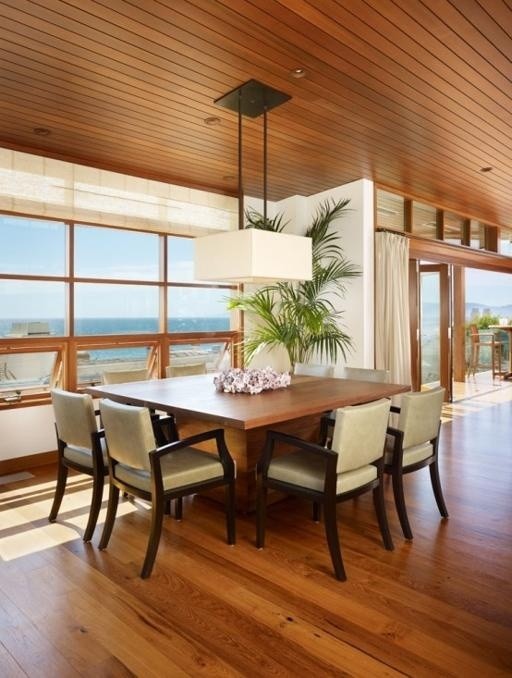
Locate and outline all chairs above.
[311,385,450,541]
[48,386,177,544]
[98,399,240,579]
[341,366,391,386]
[250,397,396,579]
[293,363,334,378]
[464,324,505,385]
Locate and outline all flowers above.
[211,365,292,395]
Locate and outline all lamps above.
[196,82,315,284]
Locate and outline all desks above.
[488,321,512,381]
[86,367,413,553]
